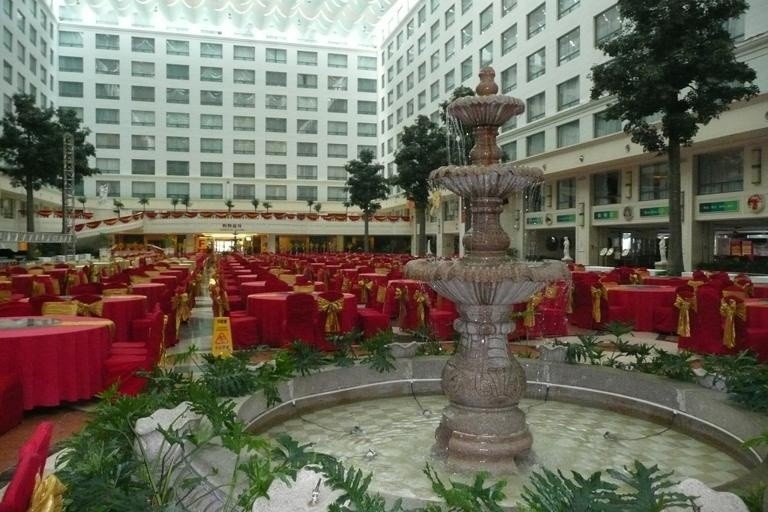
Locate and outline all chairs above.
[0,248,766,363]
[1,418,70,510]
[102,303,161,406]
[2,373,27,435]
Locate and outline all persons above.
[243,245,260,255]
[562,236,571,259]
[658,234,667,262]
